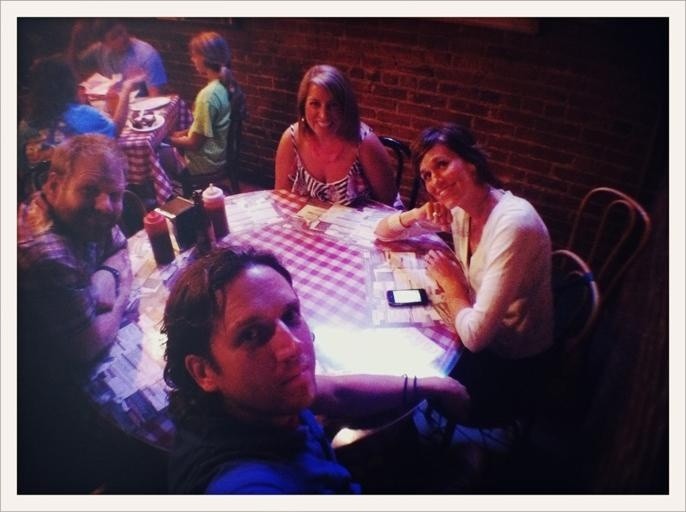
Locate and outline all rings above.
[432,211,441,218]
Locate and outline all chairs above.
[526,181,654,380]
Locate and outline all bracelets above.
[94,265,122,298]
[412,375,417,403]
[399,374,408,405]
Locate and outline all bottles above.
[202,182,229,239]
[144,210,176,265]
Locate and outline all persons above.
[15,54,141,164]
[273,64,397,207]
[17,131,133,387]
[69,20,166,99]
[374,120,557,368]
[165,30,246,196]
[158,240,474,493]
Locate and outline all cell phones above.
[386,288,428,307]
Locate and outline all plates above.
[127,114,164,132]
